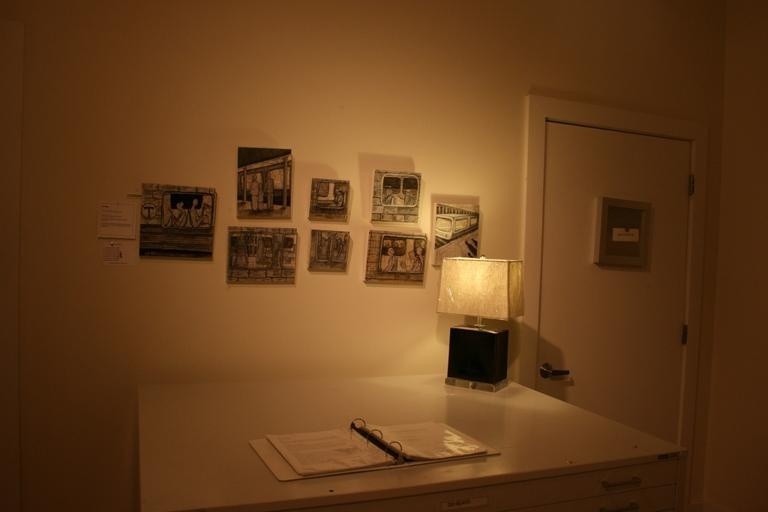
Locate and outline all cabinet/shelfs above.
[131,368,690,512]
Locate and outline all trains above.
[435,212,478,240]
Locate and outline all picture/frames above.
[590,194,654,270]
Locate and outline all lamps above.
[437,254,527,391]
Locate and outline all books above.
[249,418,501,481]
